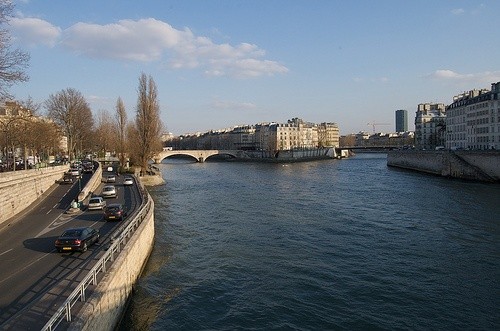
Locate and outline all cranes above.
[368,120,392,133]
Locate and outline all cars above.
[104,203,128,221]
[55,226,100,253]
[107,175,115,182]
[64,160,94,183]
[88,196,106,211]
[123,178,133,186]
[108,167,113,172]
[103,186,119,199]
[16,164,31,170]
